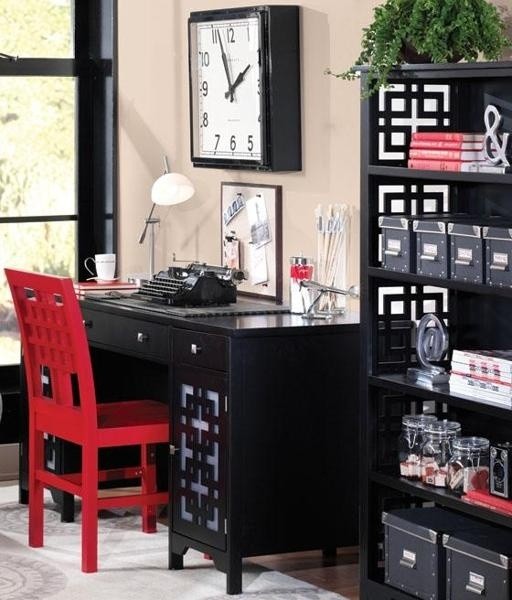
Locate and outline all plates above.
[87,277,120,284]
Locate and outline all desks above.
[18,277,359,593]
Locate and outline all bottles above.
[397,414,490,495]
[289,255,312,315]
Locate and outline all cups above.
[84,254,116,278]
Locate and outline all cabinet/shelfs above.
[361,59,510,600]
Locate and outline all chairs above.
[2,265,175,578]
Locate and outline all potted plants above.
[332,0,512,98]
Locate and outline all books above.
[448,348,512,408]
[72,281,140,296]
[460,489,512,517]
[407,132,507,175]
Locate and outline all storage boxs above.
[449,222,484,283]
[414,220,447,279]
[381,506,439,594]
[484,225,511,291]
[442,517,509,594]
[376,216,411,274]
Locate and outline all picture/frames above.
[218,180,283,304]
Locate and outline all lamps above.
[138,154,198,280]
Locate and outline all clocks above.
[187,6,300,174]
